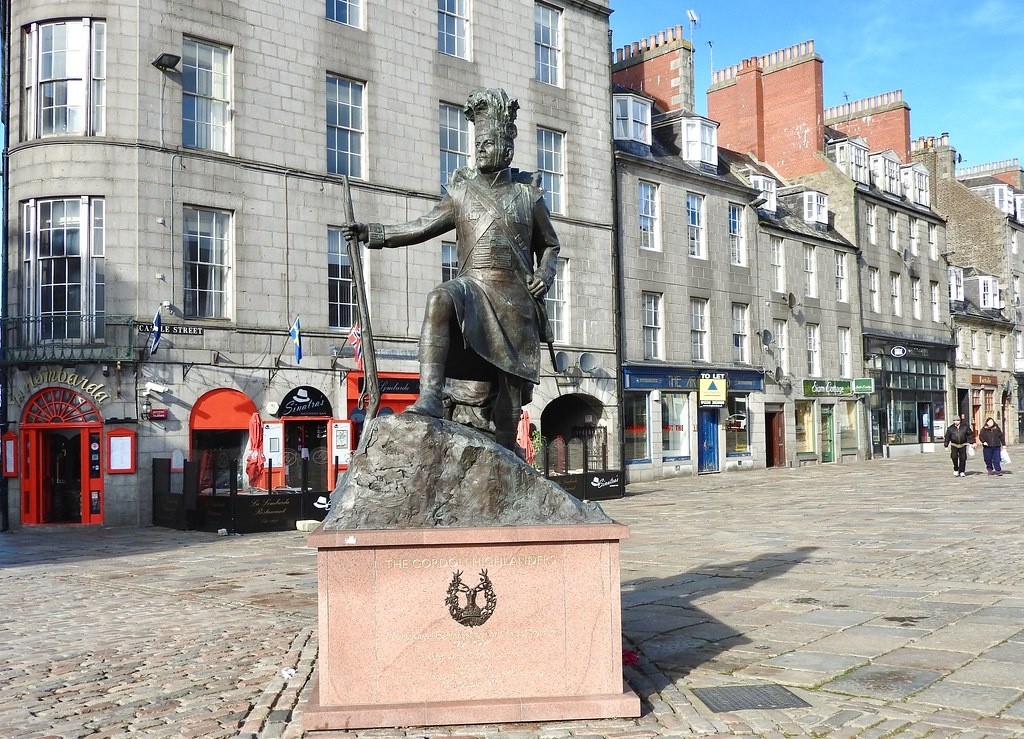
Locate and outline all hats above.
[953,415,961,420]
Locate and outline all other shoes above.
[996,470,1003,476]
[987,469,993,475]
[953,471,959,476]
[960,472,965,477]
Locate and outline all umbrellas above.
[246,413,266,494]
[518,410,535,467]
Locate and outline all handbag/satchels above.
[1000,447,1012,465]
[968,443,976,456]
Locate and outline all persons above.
[944,415,974,477]
[341,88,560,463]
[979,417,1011,476]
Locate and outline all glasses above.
[953,420,960,421]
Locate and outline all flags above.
[150,306,162,355]
[348,322,363,370]
[289,316,302,364]
